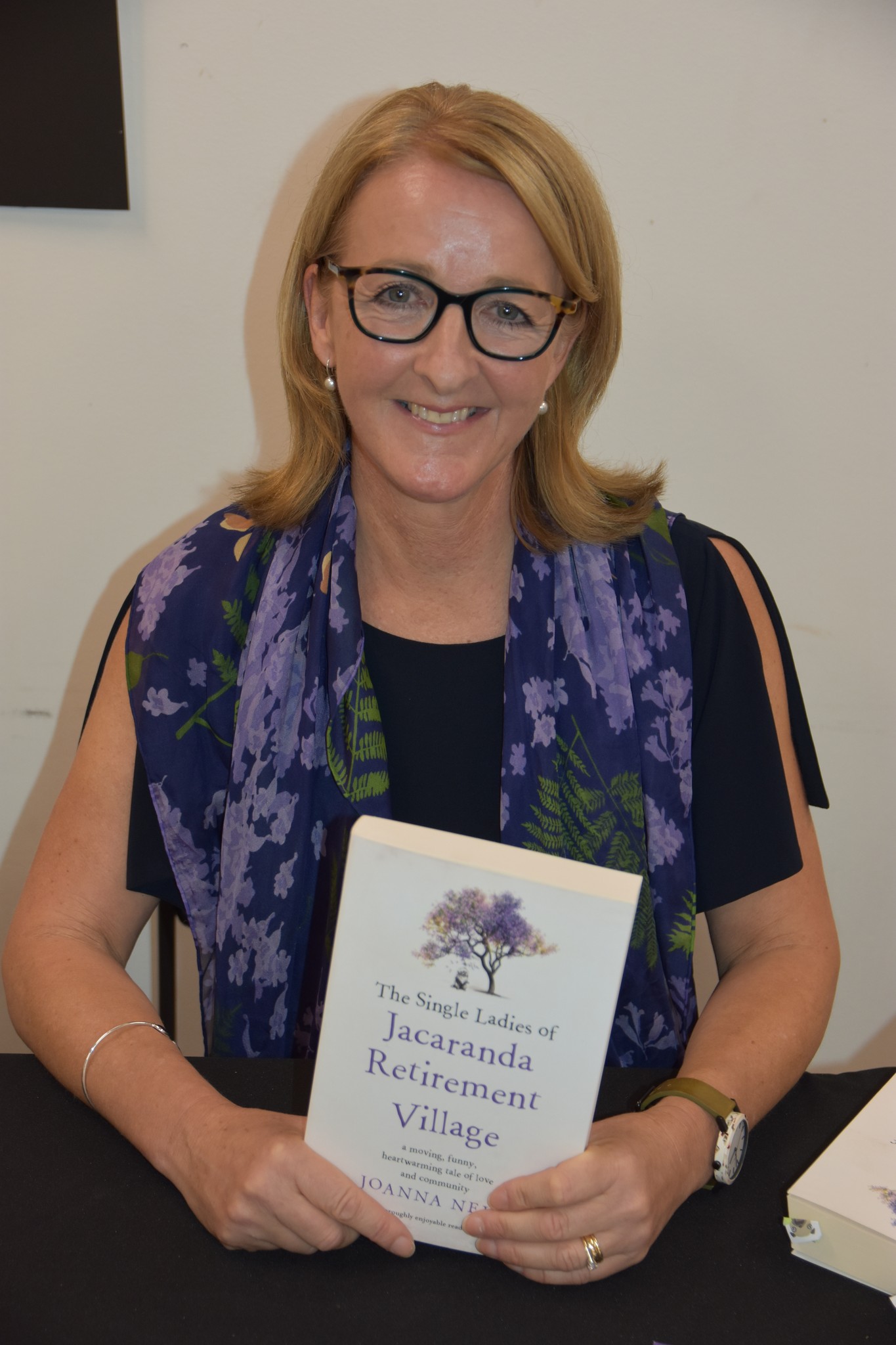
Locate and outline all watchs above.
[633,1077,749,1190]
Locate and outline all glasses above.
[322,255,582,361]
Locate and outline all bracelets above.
[81,1020,180,1113]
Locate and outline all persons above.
[0,82,842,1345]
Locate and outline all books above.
[306,814,644,1254]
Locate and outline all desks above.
[0,1055,896,1345]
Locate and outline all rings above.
[579,1234,603,1271]
